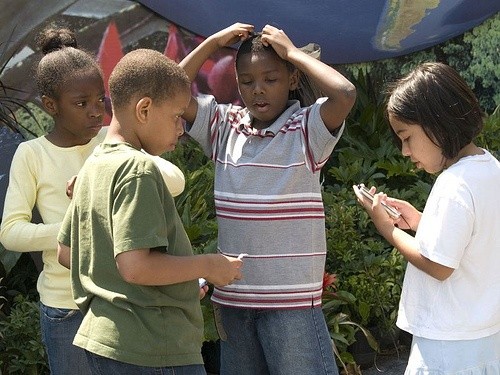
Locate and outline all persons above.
[0,22,185,375]
[176,23,356,375]
[57,49,244,375]
[353,62,500,375]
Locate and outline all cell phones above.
[358,184,401,220]
